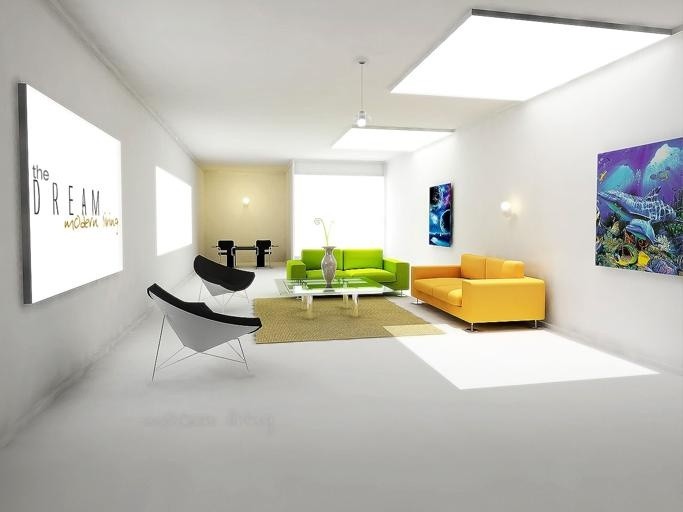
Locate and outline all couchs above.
[286,247,409,298]
[409,253,547,334]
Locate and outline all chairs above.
[216,239,237,267]
[254,240,271,267]
[147,254,262,382]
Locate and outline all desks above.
[226,245,271,268]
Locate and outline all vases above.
[320,246,337,284]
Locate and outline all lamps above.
[355,61,366,127]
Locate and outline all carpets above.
[251,296,447,345]
